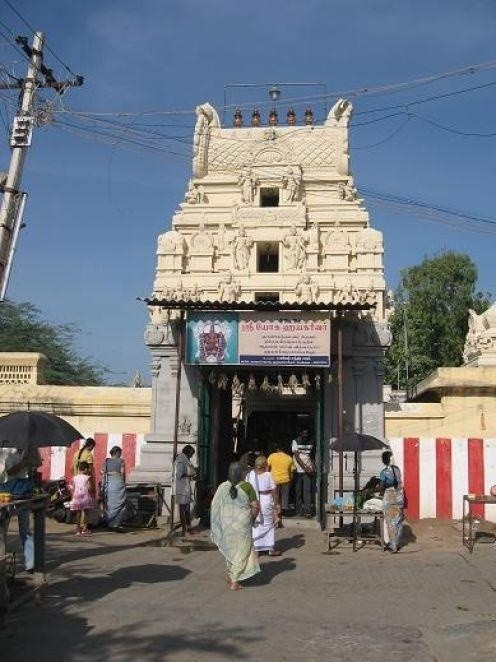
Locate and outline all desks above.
[463,494,496,553]
[324,511,386,552]
[0,492,51,615]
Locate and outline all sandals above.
[224,574,244,591]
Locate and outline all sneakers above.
[269,551,280,556]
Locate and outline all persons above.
[282,226,305,271]
[296,274,318,304]
[283,166,299,203]
[209,461,261,590]
[5,448,42,548]
[246,456,283,557]
[245,437,267,468]
[171,444,200,536]
[160,284,202,302]
[292,428,317,520]
[217,272,241,302]
[380,450,409,552]
[70,437,128,537]
[267,442,296,515]
[339,282,377,305]
[239,167,258,205]
[231,224,253,271]
[344,180,358,200]
[183,181,199,204]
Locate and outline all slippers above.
[81,529,91,533]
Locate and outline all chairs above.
[194,478,213,526]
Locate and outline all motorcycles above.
[33,474,73,524]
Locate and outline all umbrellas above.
[329,433,387,552]
[0,400,86,447]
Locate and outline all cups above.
[468,493,476,500]
[0,493,12,503]
[325,496,356,514]
